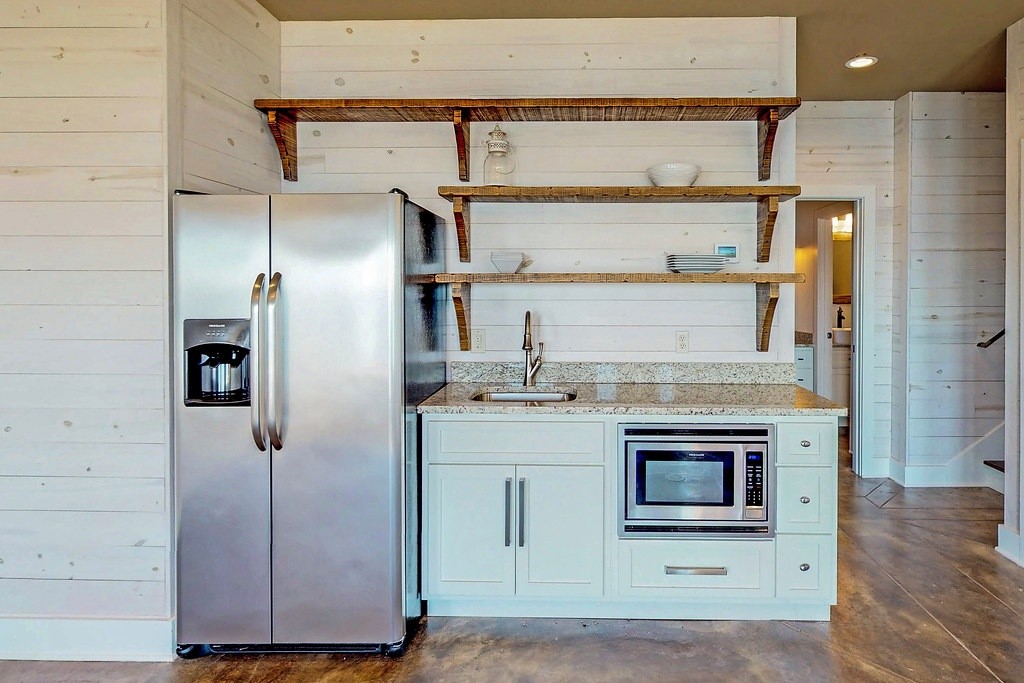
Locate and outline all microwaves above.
[617,423,776,538]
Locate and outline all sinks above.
[832,326,851,346]
[468,387,579,402]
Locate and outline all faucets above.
[522,310,546,386]
[837,306,847,327]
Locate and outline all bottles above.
[483,124,513,187]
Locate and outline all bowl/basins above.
[646,162,702,186]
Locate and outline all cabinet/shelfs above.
[418,384,848,621]
[255,97,806,352]
[795,347,813,393]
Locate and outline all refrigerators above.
[173,194,445,658]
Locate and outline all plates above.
[666,254,726,274]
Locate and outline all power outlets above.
[676,331,689,354]
